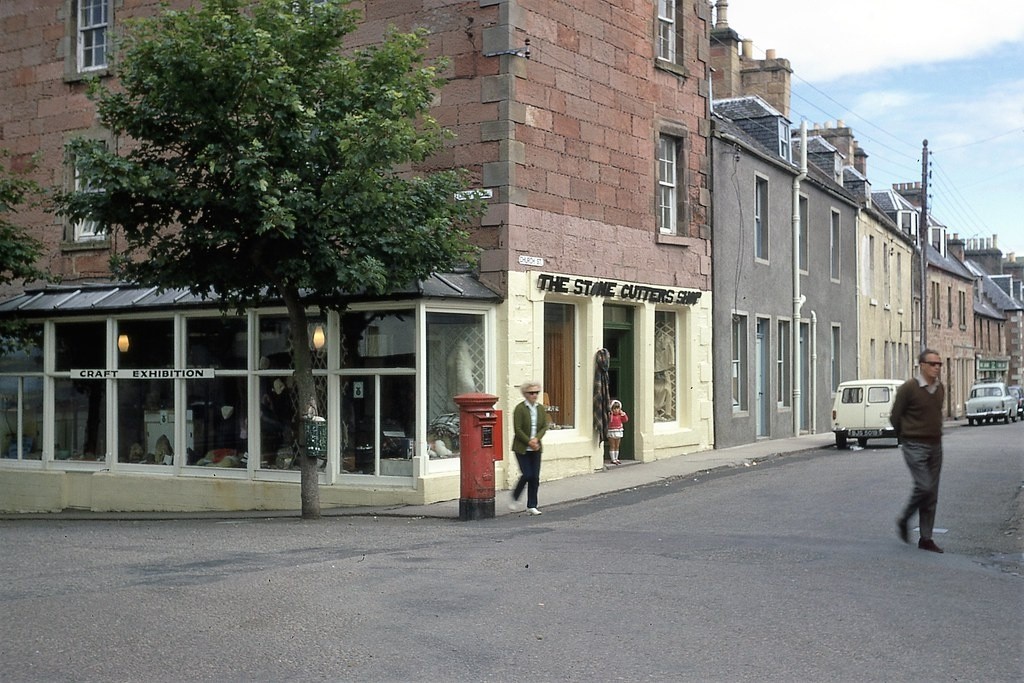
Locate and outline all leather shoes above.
[899,519,908,542]
[918,538,943,553]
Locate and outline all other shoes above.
[615,459,621,464]
[611,460,619,465]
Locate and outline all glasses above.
[923,361,943,367]
[525,391,540,395]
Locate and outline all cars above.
[963,383,1024,426]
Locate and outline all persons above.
[607,399,628,466]
[890,348,945,554]
[260,378,297,464]
[507,378,547,515]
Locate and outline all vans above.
[831,379,905,450]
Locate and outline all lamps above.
[312,322,325,358]
[117,330,130,352]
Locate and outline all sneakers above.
[527,507,542,515]
[507,496,517,510]
